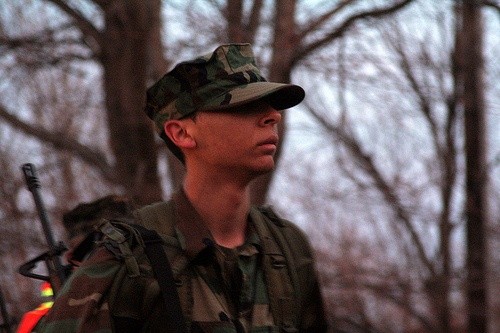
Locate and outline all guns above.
[17,162,74,300]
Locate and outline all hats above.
[62,195,133,238]
[144,43,306,135]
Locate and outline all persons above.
[32,43,330,333]
